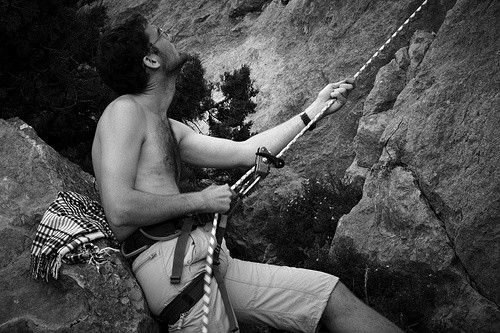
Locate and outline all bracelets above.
[300,111,317,132]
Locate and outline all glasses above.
[142,27,168,70]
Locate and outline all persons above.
[90,13,406,333]
